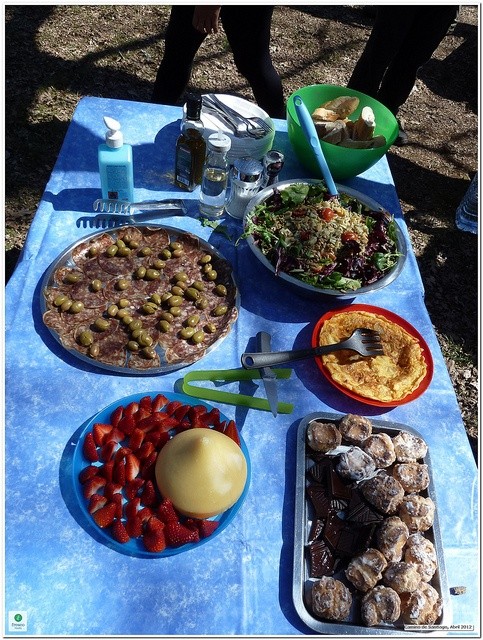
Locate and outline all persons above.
[148,7,288,120]
[344,7,460,116]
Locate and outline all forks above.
[200,93,273,133]
[241,328,384,371]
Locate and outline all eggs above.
[318,310,428,404]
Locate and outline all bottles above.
[173,95,206,192]
[262,151,285,189]
[225,158,259,220]
[199,132,231,218]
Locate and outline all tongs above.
[181,366,292,415]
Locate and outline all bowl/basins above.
[286,84,399,184]
[243,179,407,296]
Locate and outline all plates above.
[40,223,242,375]
[183,94,275,163]
[73,391,251,557]
[310,303,433,408]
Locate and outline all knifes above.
[257,331,282,418]
